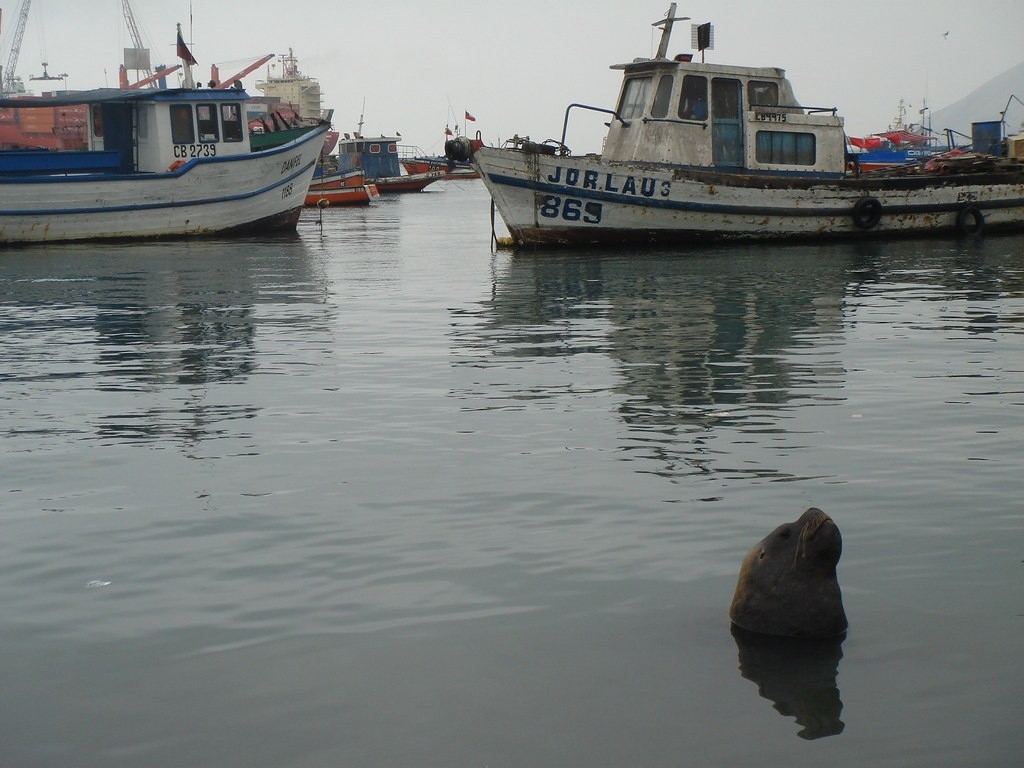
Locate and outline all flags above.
[445,129,452,134]
[466,112,475,121]
[177,33,198,66]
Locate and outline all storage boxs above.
[1006,135,1023,157]
[0,95,86,134]
[244,96,298,131]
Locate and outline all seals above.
[727,505,848,640]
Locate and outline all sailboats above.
[846,96,960,175]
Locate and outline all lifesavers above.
[954,204,987,241]
[165,160,188,172]
[852,196,882,230]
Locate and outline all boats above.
[400,109,486,179]
[308,167,365,191]
[313,96,446,192]
[0,23,331,243]
[304,184,380,206]
[445,3,1024,250]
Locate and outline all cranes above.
[211,54,275,89]
[119,1,161,89]
[119,64,182,88]
[0,48,339,155]
[0,0,63,99]
